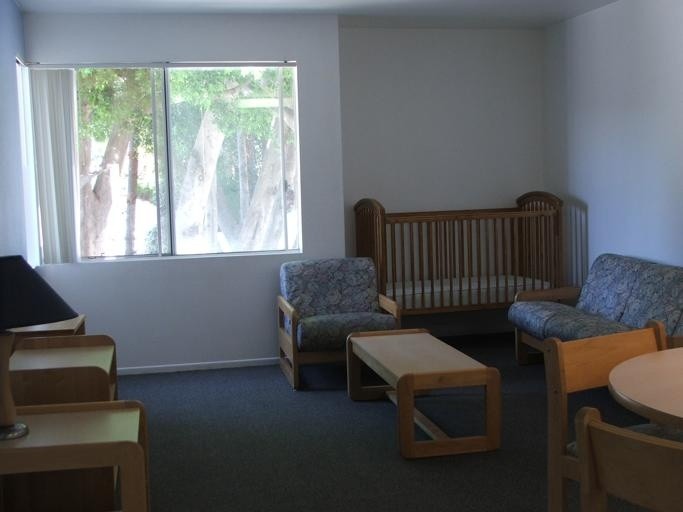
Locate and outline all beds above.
[354,191,564,328]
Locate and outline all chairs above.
[543,319,668,512]
[277,256,401,390]
[574,406,683,512]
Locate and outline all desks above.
[607,346,683,434]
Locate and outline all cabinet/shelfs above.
[8,334,118,512]
[0,400,150,512]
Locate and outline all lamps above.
[0,254,80,439]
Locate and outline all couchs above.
[508,253,683,366]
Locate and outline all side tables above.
[6,315,86,350]
